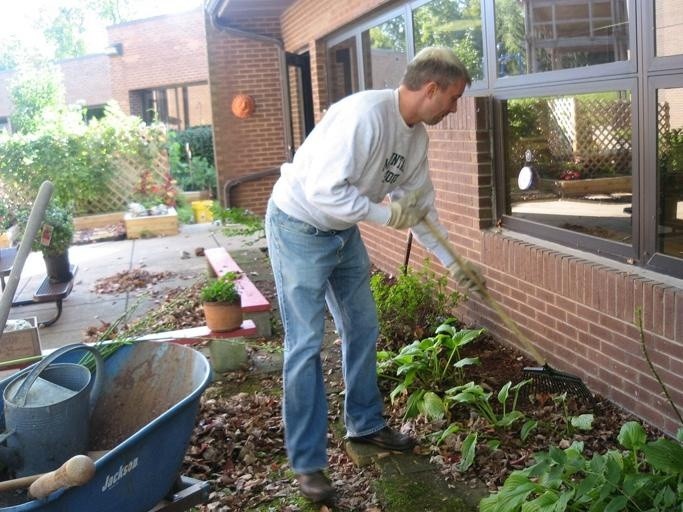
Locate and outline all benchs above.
[31,261,79,330]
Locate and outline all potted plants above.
[14,206,76,282]
[197,272,244,334]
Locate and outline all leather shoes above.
[348,425,414,451]
[296,470,335,500]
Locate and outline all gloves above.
[386,186,431,232]
[449,256,488,299]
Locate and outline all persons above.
[265,46,488,503]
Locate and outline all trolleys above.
[0,338,216,512]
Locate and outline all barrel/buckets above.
[191,199,214,225]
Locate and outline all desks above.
[0,248,16,308]
